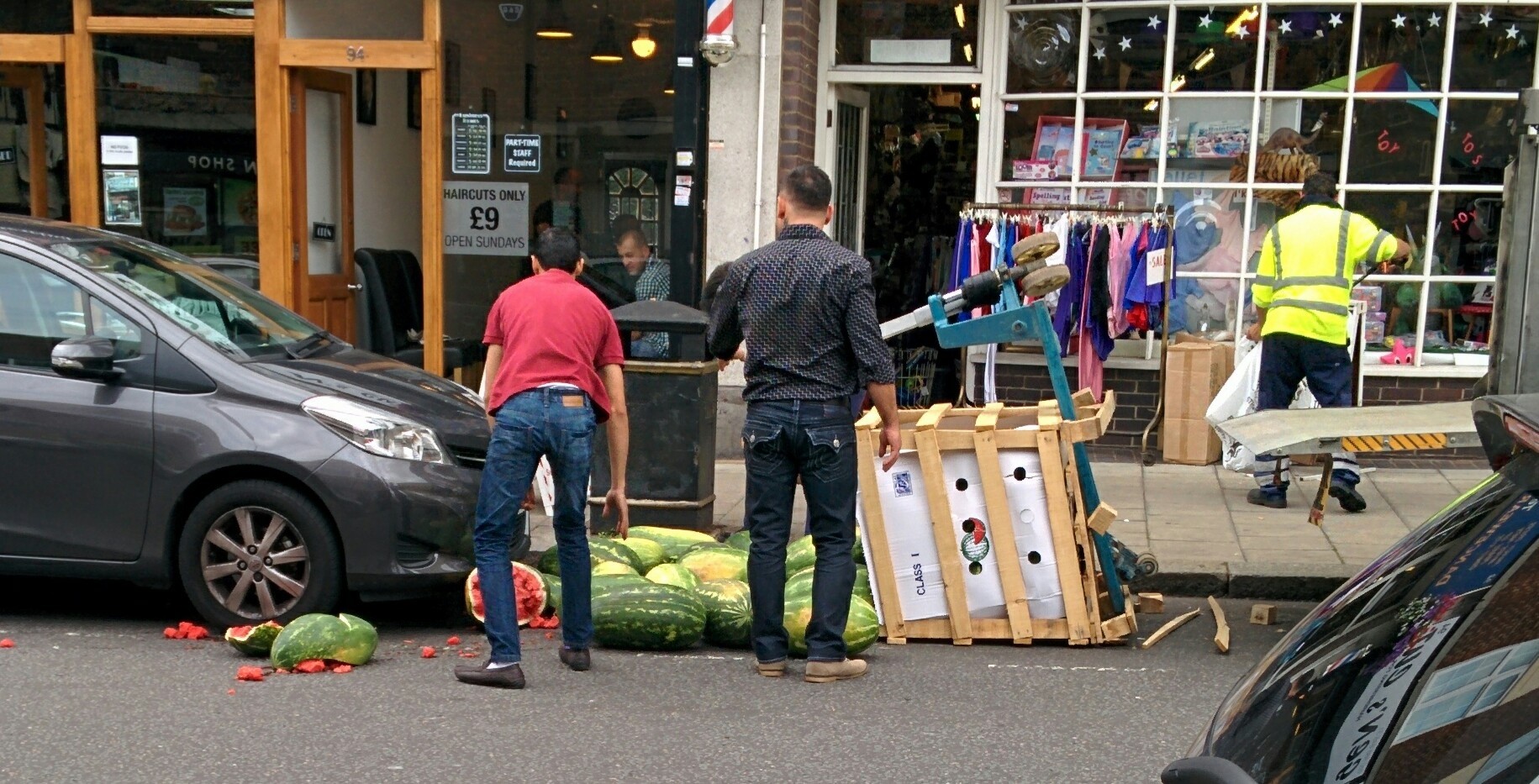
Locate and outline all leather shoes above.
[1328,480,1367,512]
[1246,488,1288,509]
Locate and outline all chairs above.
[354,247,485,389]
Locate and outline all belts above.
[801,394,853,409]
[523,387,585,397]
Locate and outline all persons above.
[520,167,584,278]
[699,261,864,537]
[1245,173,1410,511]
[454,227,629,685]
[709,164,902,682]
[610,214,670,358]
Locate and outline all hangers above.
[957,201,1174,237]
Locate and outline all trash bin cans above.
[587,299,719,536]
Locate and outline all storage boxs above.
[856,428,1069,624]
[1159,331,1233,466]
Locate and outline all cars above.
[0,210,533,635]
[185,250,261,292]
[1160,389,1539,784]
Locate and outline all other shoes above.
[558,643,591,670]
[805,658,868,682]
[752,658,785,677]
[453,660,527,689]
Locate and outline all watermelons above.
[162,526,881,683]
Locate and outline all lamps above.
[538,0,574,39]
[629,7,677,57]
[588,4,629,62]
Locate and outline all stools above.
[1460,303,1493,343]
